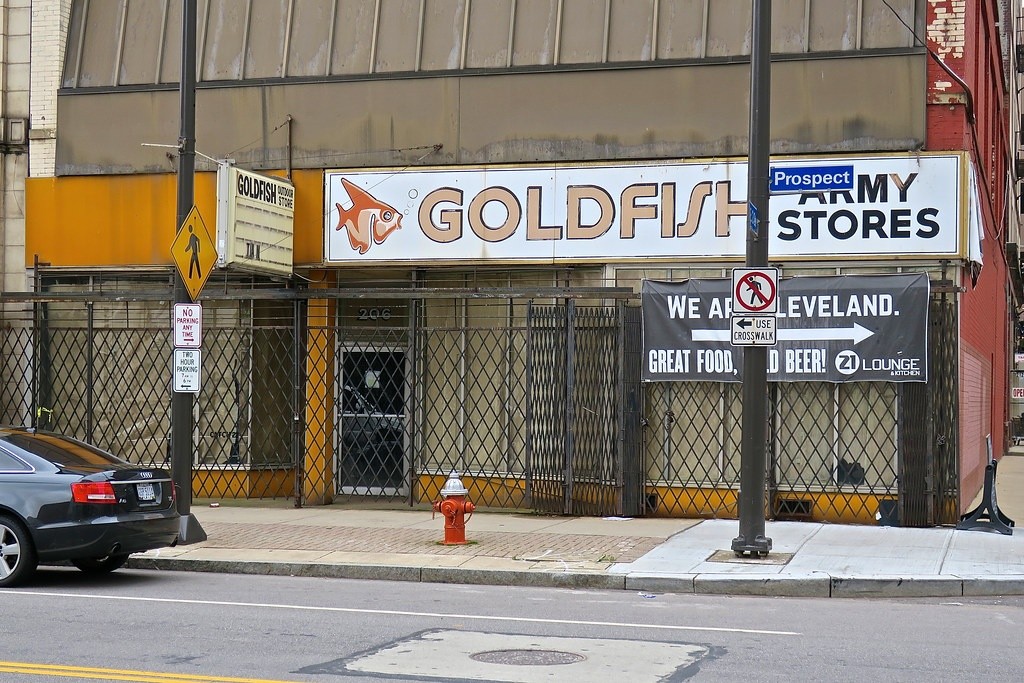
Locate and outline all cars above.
[0,426,182,587]
[343,385,404,443]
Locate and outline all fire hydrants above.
[432,474,476,545]
